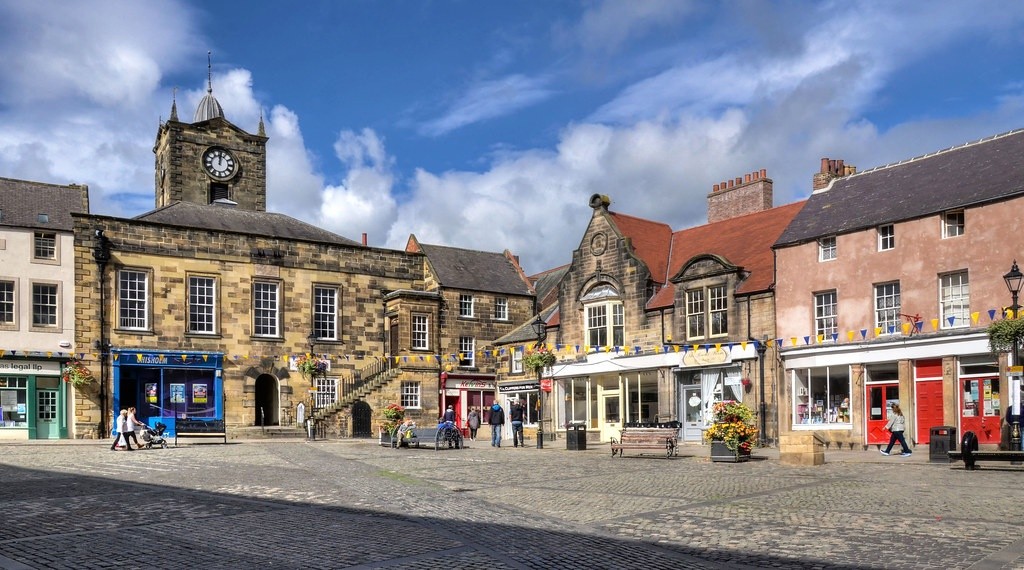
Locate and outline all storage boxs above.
[798,385,850,423]
[964,380,1000,416]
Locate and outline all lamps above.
[741,362,751,386]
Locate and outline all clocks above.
[201,145,241,182]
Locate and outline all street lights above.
[756,336,767,448]
[1002,258,1024,451]
[530,313,548,450]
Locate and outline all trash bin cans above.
[929,426,956,463]
[566,423,586,450]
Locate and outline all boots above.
[137,443,144,448]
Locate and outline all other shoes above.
[880,450,889,455]
[901,452,911,457]
[127,448,134,450]
[111,448,117,451]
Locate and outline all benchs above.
[174,419,228,446]
[947,431,1024,472]
[611,427,680,459]
[390,422,464,451]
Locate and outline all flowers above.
[704,400,753,460]
[62,361,93,389]
[295,356,327,379]
[380,403,406,435]
[521,343,556,371]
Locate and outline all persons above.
[467,407,481,442]
[1006,403,1024,451]
[880,403,912,457]
[488,400,505,447]
[126,407,144,448]
[110,409,135,451]
[509,399,525,448]
[443,405,455,424]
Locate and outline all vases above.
[379,424,409,448]
[710,437,751,462]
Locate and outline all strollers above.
[136,421,169,450]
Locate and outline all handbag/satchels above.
[118,433,126,446]
